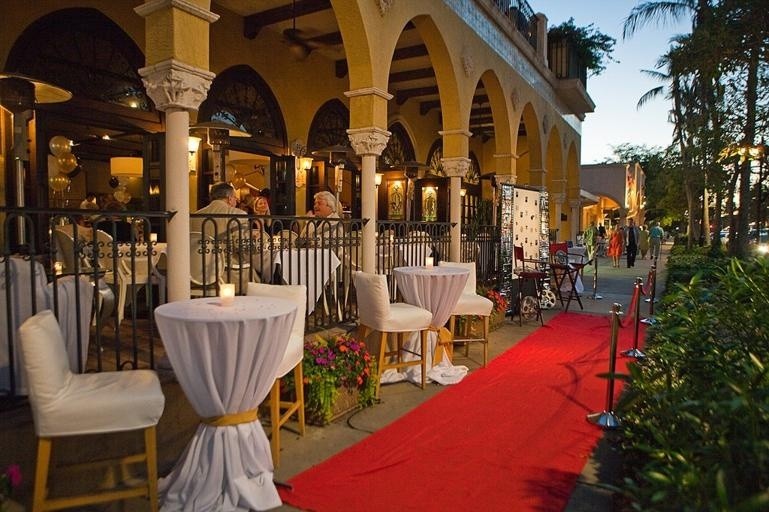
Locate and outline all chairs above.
[242,282,306,470]
[17,309,165,512]
[513,242,585,326]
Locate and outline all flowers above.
[280,330,382,428]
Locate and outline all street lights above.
[726,139,762,249]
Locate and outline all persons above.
[79,189,101,216]
[246,194,274,227]
[298,189,347,257]
[583,217,668,272]
[190,182,251,267]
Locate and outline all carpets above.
[277,311,650,512]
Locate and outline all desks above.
[150,295,298,511]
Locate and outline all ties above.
[315,220,322,228]
